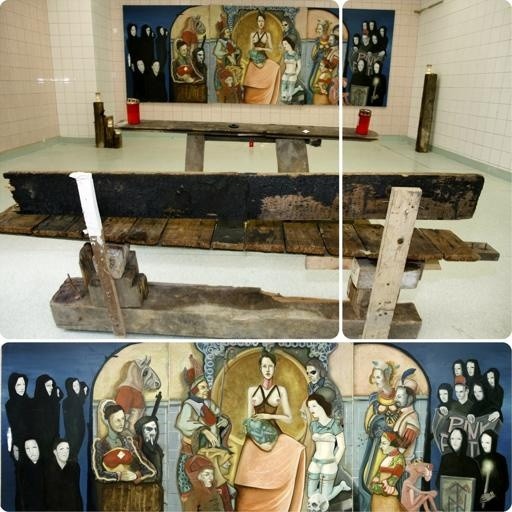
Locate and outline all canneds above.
[126,98,140,125]
[355,109,371,135]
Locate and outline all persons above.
[134,416,164,483]
[93,405,155,485]
[213,22,241,104]
[233,351,308,511]
[435,358,509,511]
[243,13,281,104]
[362,359,421,511]
[5,372,86,512]
[278,15,304,104]
[308,13,388,107]
[301,359,350,510]
[174,363,233,511]
[126,18,208,102]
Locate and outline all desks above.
[111,120,378,173]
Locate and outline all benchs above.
[0,174,500,339]
